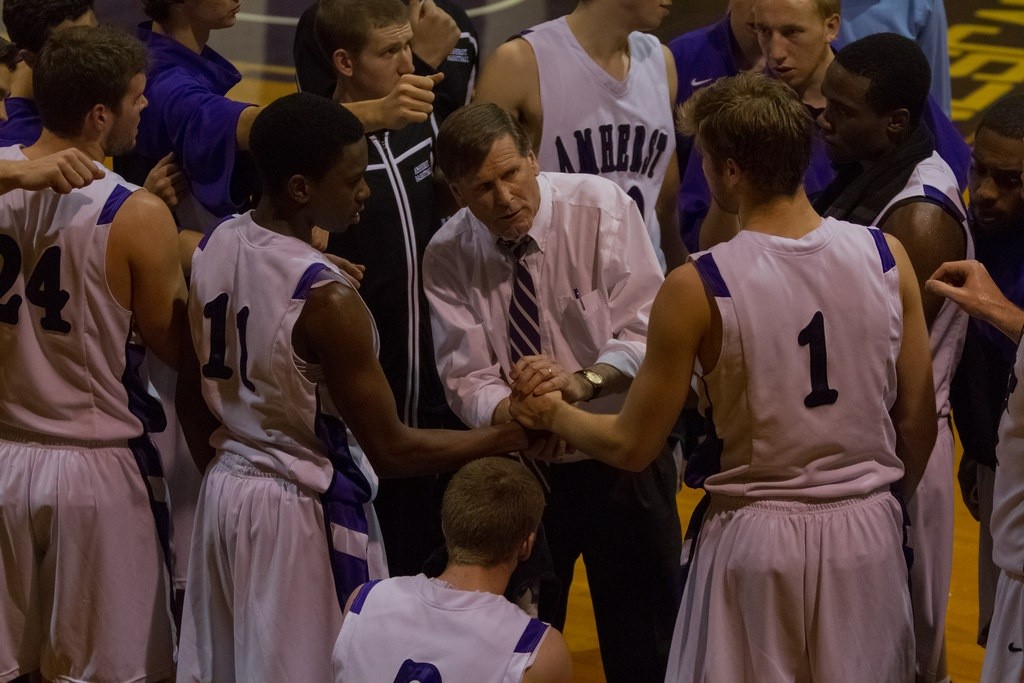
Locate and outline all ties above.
[495,234,541,366]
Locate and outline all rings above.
[547,368,552,377]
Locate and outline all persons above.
[423,101,684,683]
[0,0,1024,683]
[330,458,573,683]
[509,71,939,683]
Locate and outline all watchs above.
[574,370,604,403]
